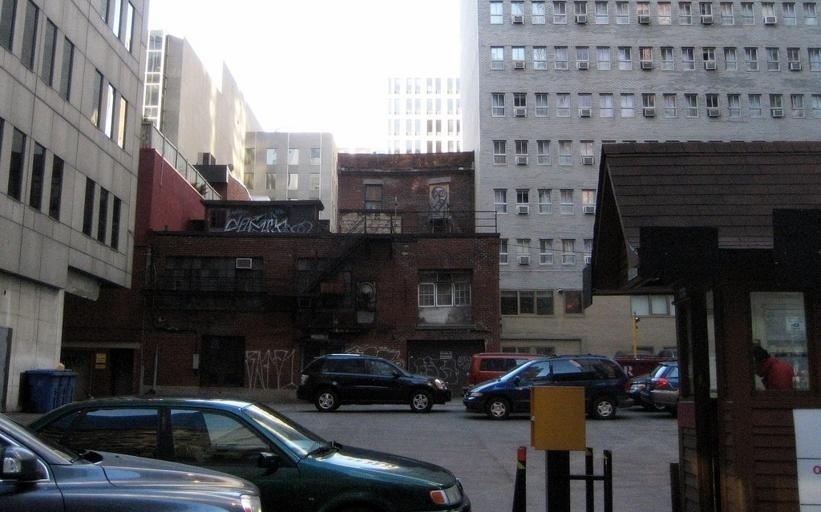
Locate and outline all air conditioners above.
[583,206,596,216]
[643,108,656,116]
[517,155,527,164]
[772,109,785,117]
[707,108,721,117]
[704,61,717,70]
[514,63,525,69]
[763,16,778,24]
[581,157,594,165]
[512,15,526,23]
[576,15,587,24]
[641,61,653,69]
[639,15,651,24]
[585,256,592,264]
[515,108,526,116]
[578,63,588,68]
[700,14,714,24]
[235,257,252,271]
[519,207,529,214]
[518,255,530,266]
[581,109,591,117]
[791,62,802,71]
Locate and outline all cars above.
[27,395,472,511]
[0,413,262,511]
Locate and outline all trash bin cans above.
[20,369,79,413]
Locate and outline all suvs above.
[296,353,451,413]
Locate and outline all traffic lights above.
[633,315,640,332]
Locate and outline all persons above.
[751,345,796,389]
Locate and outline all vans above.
[462,351,679,420]
